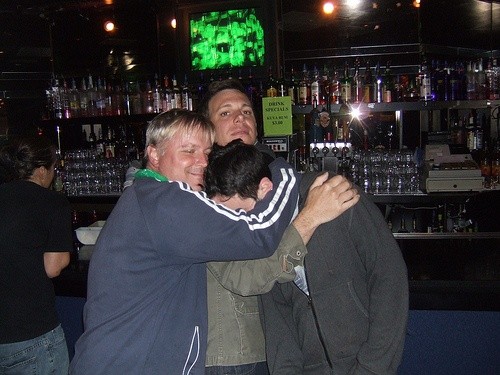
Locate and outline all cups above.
[349,150,420,193]
[63,149,127,195]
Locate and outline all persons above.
[203,138,409,375]
[196,80,361,375]
[69,108,299,375]
[0,141,75,375]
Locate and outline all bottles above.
[297,94,395,170]
[388,203,477,233]
[449,113,484,153]
[297,62,500,105]
[48,64,299,119]
[82,128,146,160]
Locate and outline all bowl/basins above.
[74,221,107,245]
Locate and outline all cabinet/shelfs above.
[36,99,500,240]
